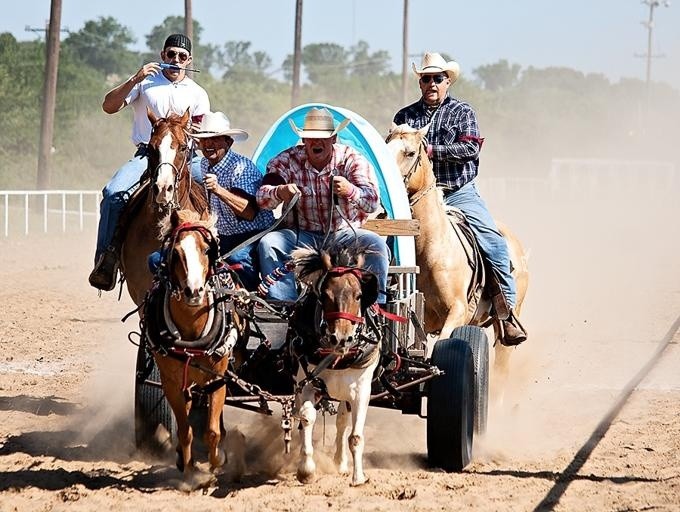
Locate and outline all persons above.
[145,110,275,293]
[85,32,211,293]
[390,49,528,349]
[255,105,394,311]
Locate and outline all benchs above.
[271,218,421,358]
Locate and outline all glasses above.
[420,74,447,84]
[166,51,187,61]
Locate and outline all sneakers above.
[89,264,114,290]
[498,318,527,346]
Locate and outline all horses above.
[385,120,530,412]
[286,237,385,487]
[144,206,240,484]
[118,105,213,332]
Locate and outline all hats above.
[287,107,352,140]
[163,33,191,56]
[182,111,249,143]
[411,52,460,88]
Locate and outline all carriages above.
[134,102,490,489]
